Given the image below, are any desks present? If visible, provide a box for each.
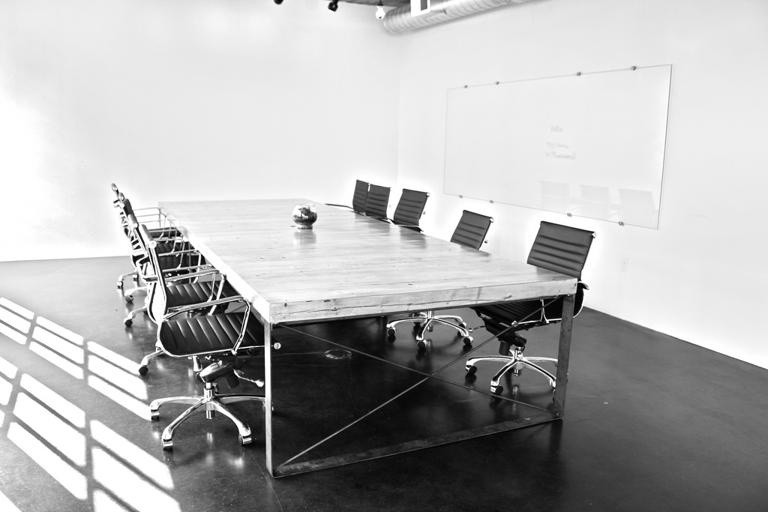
[156,200,577,479]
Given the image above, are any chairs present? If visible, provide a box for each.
[327,180,429,234]
[465,220,594,395]
[383,207,495,351]
[110,180,240,382]
[109,179,594,454]
[140,226,277,449]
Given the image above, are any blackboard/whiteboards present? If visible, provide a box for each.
[442,63,671,230]
